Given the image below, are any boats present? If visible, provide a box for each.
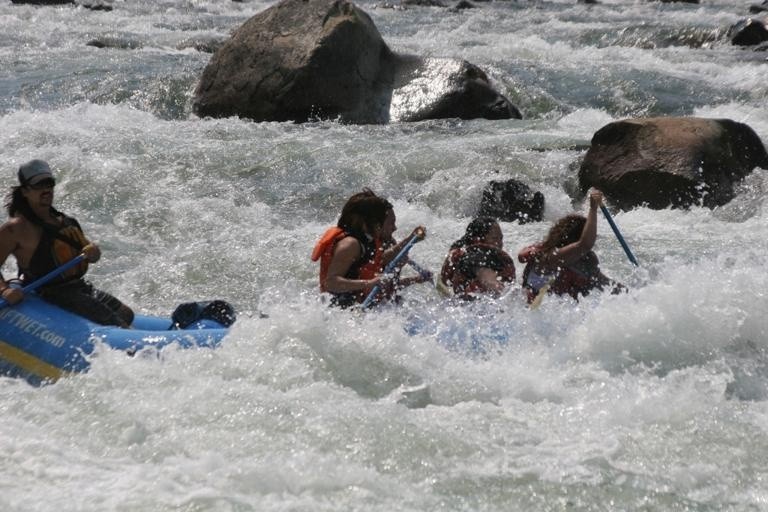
[0,276,507,388]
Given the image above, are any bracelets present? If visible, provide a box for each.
[1,286,9,295]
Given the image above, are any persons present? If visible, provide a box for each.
[0,159,135,330]
[516,188,628,310]
[361,196,434,304]
[442,217,505,296]
[310,186,423,311]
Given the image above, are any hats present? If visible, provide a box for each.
[17,160,56,185]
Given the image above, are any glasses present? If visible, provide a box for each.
[27,179,55,190]
